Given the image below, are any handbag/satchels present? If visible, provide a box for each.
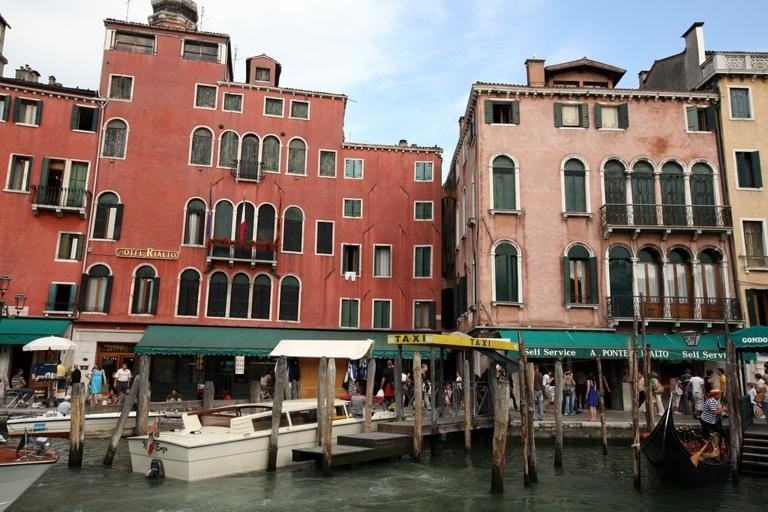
[425,382,429,390]
[653,383,662,393]
[673,387,682,396]
[753,391,764,404]
[566,390,570,395]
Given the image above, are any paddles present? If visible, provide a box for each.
[689,437,712,466]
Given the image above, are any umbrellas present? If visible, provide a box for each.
[21,334,80,383]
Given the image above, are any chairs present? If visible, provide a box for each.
[2,376,32,409]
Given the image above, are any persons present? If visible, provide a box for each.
[342,355,464,411]
[112,363,133,397]
[166,387,182,402]
[637,366,768,449]
[64,362,81,395]
[55,394,71,415]
[89,361,106,406]
[84,366,93,405]
[55,360,67,378]
[222,390,232,400]
[10,367,34,404]
[507,365,612,421]
[109,388,117,399]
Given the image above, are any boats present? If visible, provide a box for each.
[128,397,396,481]
[7,411,166,439]
[0,437,60,512]
[637,390,731,493]
